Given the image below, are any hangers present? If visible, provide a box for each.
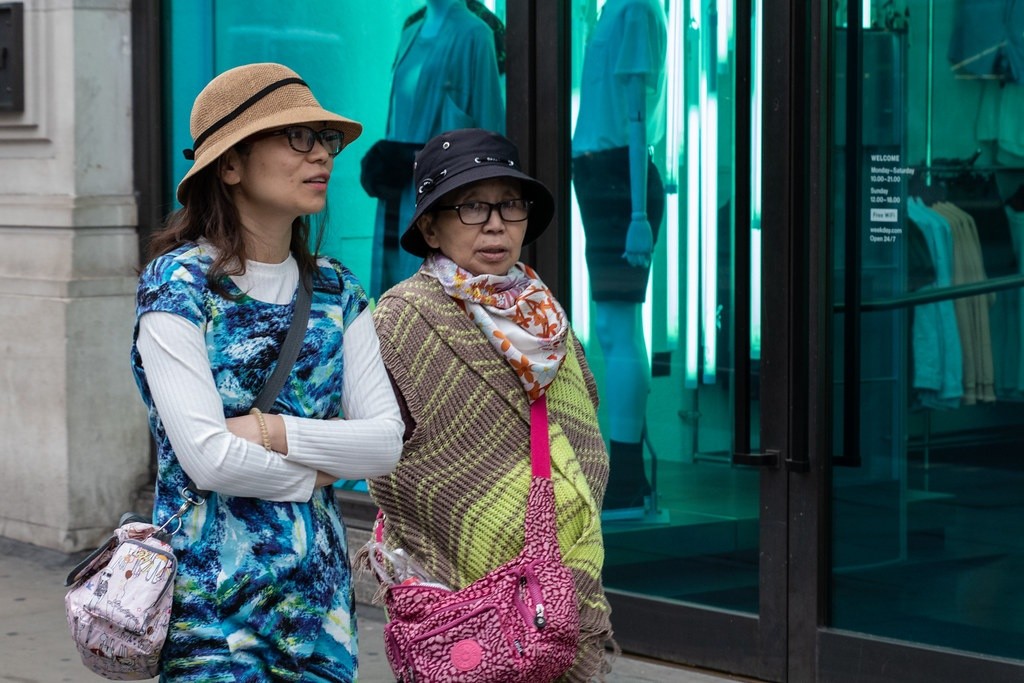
[908,164,995,209]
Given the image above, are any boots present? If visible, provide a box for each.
[596,437,650,521]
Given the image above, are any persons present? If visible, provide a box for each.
[367,133,615,683]
[567,0,672,519]
[130,62,405,683]
[373,0,503,293]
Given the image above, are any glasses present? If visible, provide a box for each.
[430,198,533,226]
[236,125,345,159]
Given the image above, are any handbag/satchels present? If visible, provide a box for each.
[357,138,425,199]
[384,548,581,683]
[64,520,178,680]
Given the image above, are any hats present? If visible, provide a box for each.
[399,128,555,259]
[177,62,364,209]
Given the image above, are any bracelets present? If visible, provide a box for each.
[250,407,271,453]
